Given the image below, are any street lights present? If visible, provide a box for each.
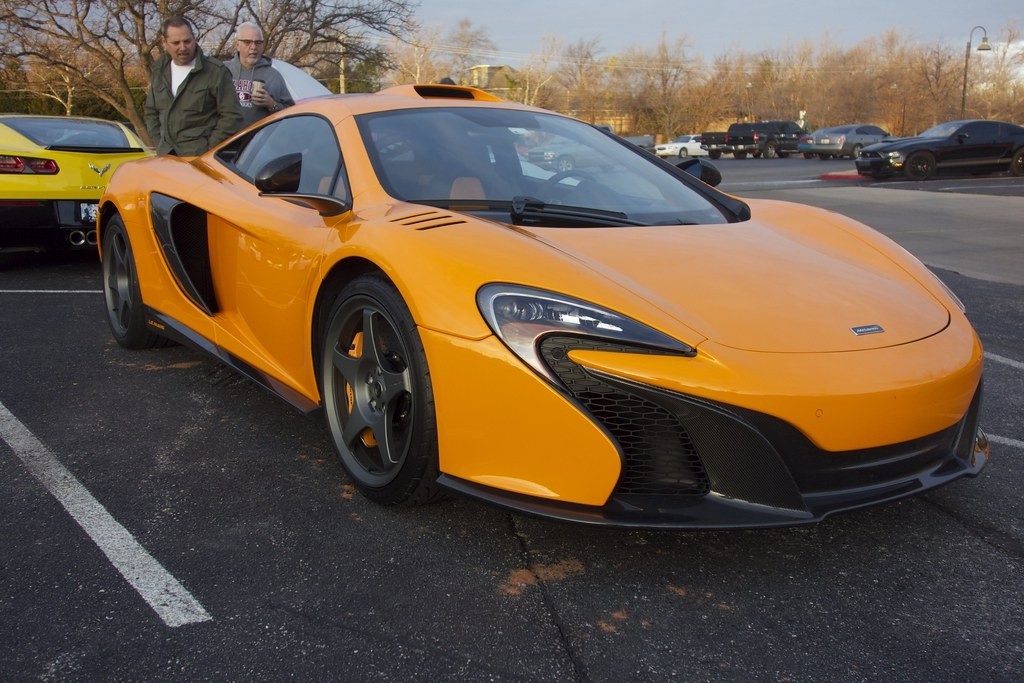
[961,25,991,119]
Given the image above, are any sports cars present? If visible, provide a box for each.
[0,111,155,259]
[97,82,991,530]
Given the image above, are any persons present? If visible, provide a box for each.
[222,22,296,127]
[145,17,242,158]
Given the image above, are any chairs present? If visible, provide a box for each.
[419,139,515,199]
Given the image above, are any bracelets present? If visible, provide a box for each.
[268,103,278,111]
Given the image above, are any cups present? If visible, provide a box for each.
[252,79,265,103]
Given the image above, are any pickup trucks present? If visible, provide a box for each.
[701,120,805,158]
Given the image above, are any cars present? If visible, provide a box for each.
[655,134,711,158]
[855,120,1024,180]
[798,123,893,160]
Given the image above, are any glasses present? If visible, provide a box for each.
[237,39,264,45]
